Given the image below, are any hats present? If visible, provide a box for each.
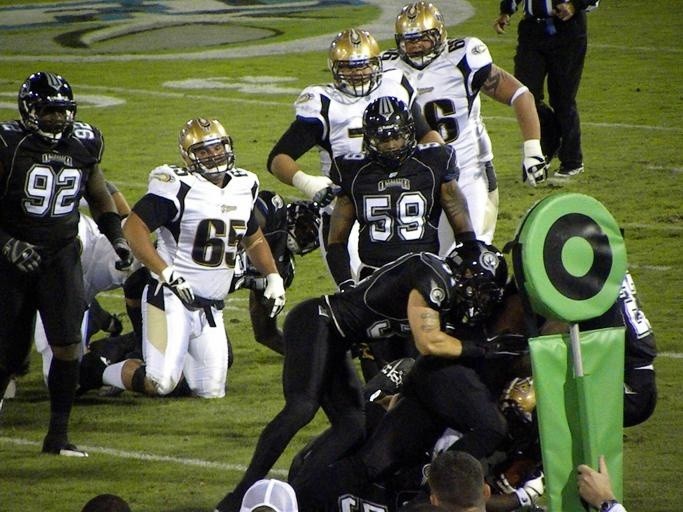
[239,479,299,512]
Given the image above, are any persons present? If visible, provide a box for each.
[207,241,509,509]
[85,183,325,383]
[267,28,453,293]
[114,115,286,400]
[570,458,631,512]
[494,1,598,176]
[321,94,476,295]
[0,73,134,459]
[427,448,494,511]
[308,345,565,512]
[380,8,549,265]
[589,232,660,429]
[37,175,147,391]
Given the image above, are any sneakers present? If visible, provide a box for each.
[89,335,129,364]
[98,386,125,399]
[541,141,562,164]
[43,436,89,458]
[552,157,585,178]
[75,354,110,398]
[216,493,240,512]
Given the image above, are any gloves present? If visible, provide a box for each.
[3,240,43,275]
[151,266,196,305]
[477,330,529,362]
[514,467,546,508]
[293,170,342,208]
[261,273,287,321]
[105,228,136,271]
[520,139,548,187]
[339,279,357,295]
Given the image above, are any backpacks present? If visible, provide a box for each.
[511,203,657,469]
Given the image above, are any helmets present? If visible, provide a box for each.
[287,201,335,256]
[363,97,417,171]
[179,118,235,175]
[18,72,78,145]
[499,376,537,435]
[395,2,447,70]
[328,30,382,97]
[445,240,508,321]
